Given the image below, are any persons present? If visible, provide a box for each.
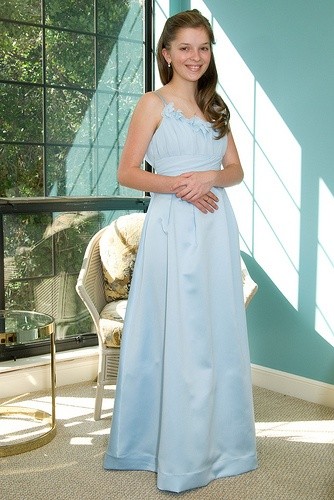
[104,6,260,495]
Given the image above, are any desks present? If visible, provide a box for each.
[0,305,60,459]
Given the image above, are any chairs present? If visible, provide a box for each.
[75,209,260,424]
